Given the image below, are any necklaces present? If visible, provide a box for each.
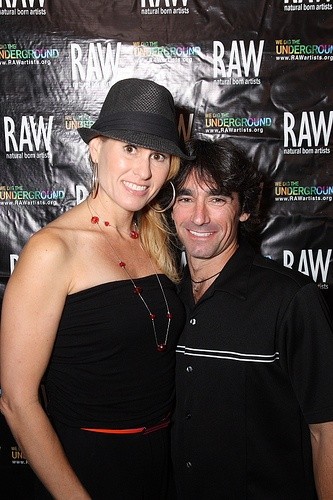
[89,196,138,239]
[86,195,173,351]
[191,272,220,292]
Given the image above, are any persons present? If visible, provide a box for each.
[156,137,333,500]
[0,78,196,500]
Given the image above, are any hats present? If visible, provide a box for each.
[76,78,195,161]
